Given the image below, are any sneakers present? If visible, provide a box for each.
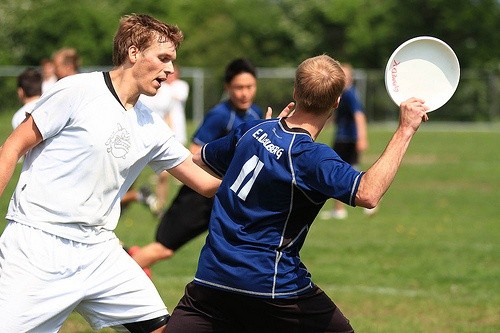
[364,207,377,214]
[335,208,347,220]
[139,187,161,216]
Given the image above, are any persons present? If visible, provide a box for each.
[119,62,190,215]
[1,13,295,333]
[320,64,378,219]
[162,55,429,333]
[123,58,266,278]
[12,47,80,132]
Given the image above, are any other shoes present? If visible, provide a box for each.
[128,244,152,277]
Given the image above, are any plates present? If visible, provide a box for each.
[384,37,460,113]
